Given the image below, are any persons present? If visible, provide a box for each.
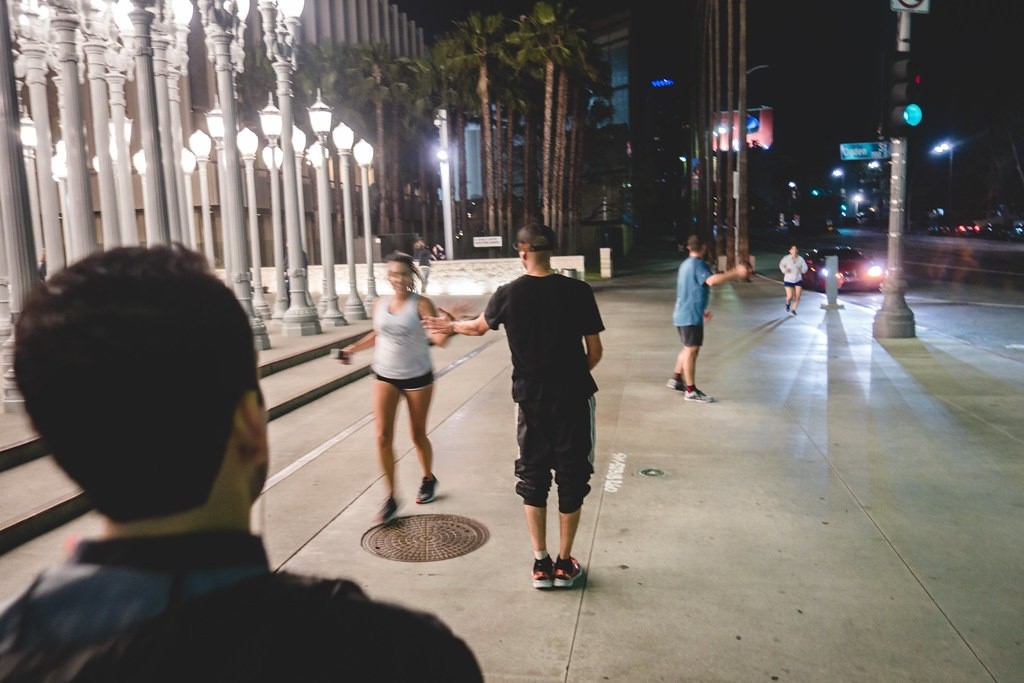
[340,250,451,520]
[419,246,437,293]
[779,244,808,315]
[283,240,308,307]
[421,223,606,589]
[666,234,748,403]
[0,239,487,683]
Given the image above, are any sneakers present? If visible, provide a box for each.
[666,379,687,392]
[553,557,582,587]
[377,497,401,522]
[684,390,714,403]
[416,473,439,504]
[532,559,556,588]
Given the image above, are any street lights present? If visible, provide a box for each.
[257,0,324,337]
[935,141,953,226]
[350,138,382,304]
[197,0,273,351]
[237,127,270,320]
[290,123,318,314]
[186,128,216,274]
[432,108,456,261]
[330,120,368,321]
[0,0,309,339]
[305,86,347,328]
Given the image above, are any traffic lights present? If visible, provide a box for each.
[882,50,924,139]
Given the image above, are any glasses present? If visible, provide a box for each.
[512,240,518,250]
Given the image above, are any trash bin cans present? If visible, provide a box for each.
[563,268,577,277]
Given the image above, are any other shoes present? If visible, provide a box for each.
[786,303,790,312]
[789,310,797,317]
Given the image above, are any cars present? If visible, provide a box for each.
[800,244,884,294]
